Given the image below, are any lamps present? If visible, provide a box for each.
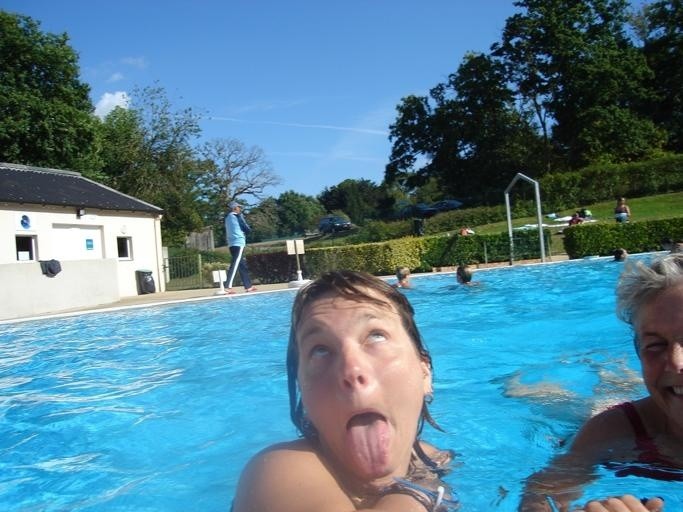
[76,208,86,217]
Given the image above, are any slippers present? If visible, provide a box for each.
[224,287,256,295]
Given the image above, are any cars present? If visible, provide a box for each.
[402,200,462,218]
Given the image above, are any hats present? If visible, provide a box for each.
[227,202,242,208]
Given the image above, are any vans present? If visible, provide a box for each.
[318,217,352,233]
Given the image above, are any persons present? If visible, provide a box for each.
[613,248,628,262]
[568,213,583,225]
[230,269,456,512]
[391,267,414,288]
[661,237,683,253]
[224,201,257,295]
[613,197,631,223]
[456,265,480,286]
[517,250,683,512]
